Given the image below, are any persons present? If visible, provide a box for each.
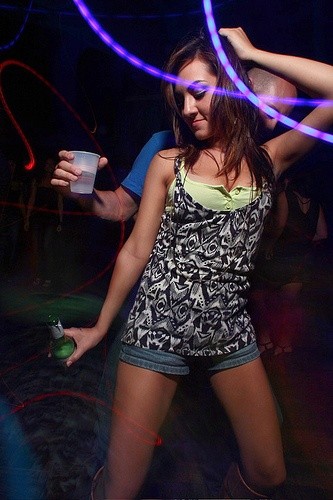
[0,66,333,460]
[49,27,333,500]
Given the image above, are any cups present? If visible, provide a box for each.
[69,151,101,194]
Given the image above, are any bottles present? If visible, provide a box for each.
[47,313,77,365]
